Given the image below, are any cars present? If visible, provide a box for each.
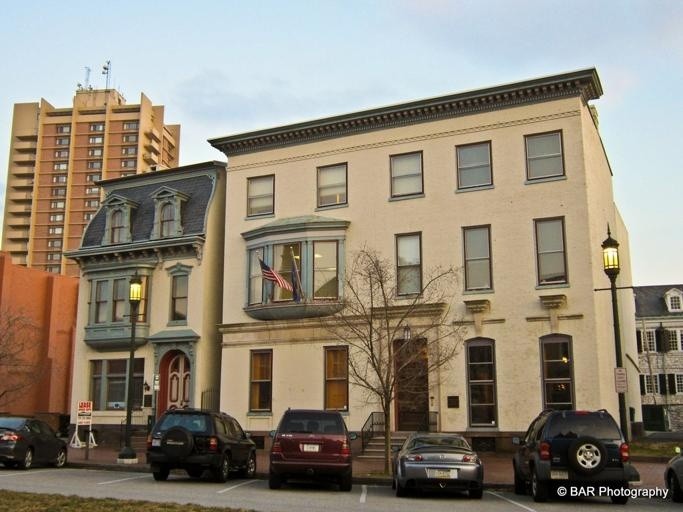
[0,413,67,468]
[388,432,487,500]
[663,446,683,500]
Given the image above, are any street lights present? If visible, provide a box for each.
[601,224,642,483]
[112,268,141,466]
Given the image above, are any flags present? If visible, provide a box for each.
[289,258,306,304]
[257,257,294,293]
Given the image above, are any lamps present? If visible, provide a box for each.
[402,323,411,342]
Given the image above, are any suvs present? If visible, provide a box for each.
[141,406,261,483]
[267,406,356,490]
[511,408,633,504]
